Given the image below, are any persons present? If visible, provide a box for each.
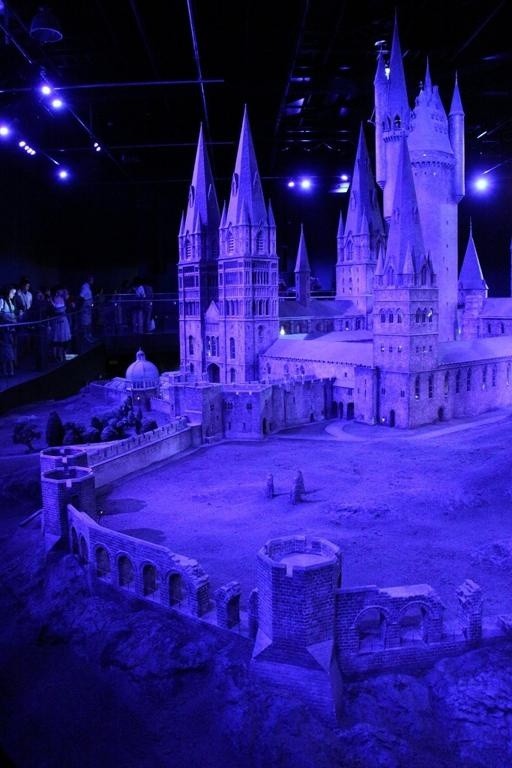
[265,471,277,500]
[1,271,158,373]
[289,465,307,506]
